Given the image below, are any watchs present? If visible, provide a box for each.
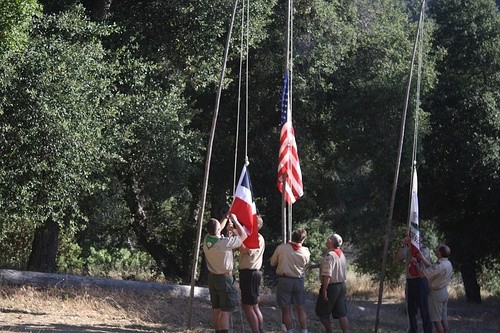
[413,262,418,266]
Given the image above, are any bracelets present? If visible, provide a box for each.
[232,219,238,225]
[399,241,404,248]
[225,213,230,219]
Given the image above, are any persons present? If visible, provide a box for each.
[227,215,266,333]
[306,233,350,333]
[396,232,433,333]
[203,208,248,333]
[270,228,310,333]
[410,244,453,333]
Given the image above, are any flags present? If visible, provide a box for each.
[227,160,260,249]
[407,161,423,276]
[277,68,304,205]
